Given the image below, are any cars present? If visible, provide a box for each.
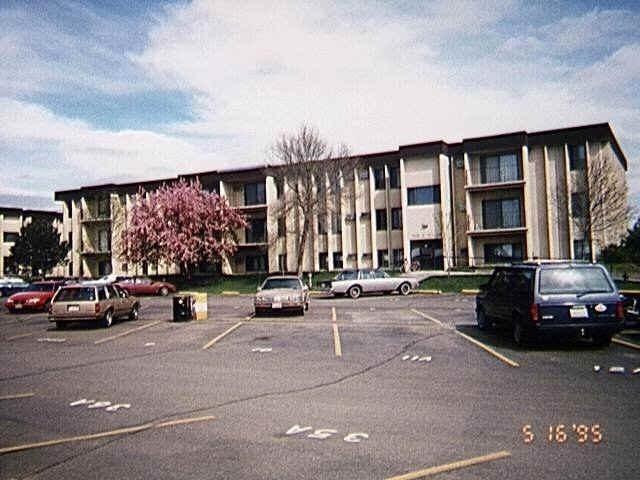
[254,276,309,316]
[0,277,176,329]
[317,269,417,298]
[475,262,626,346]
